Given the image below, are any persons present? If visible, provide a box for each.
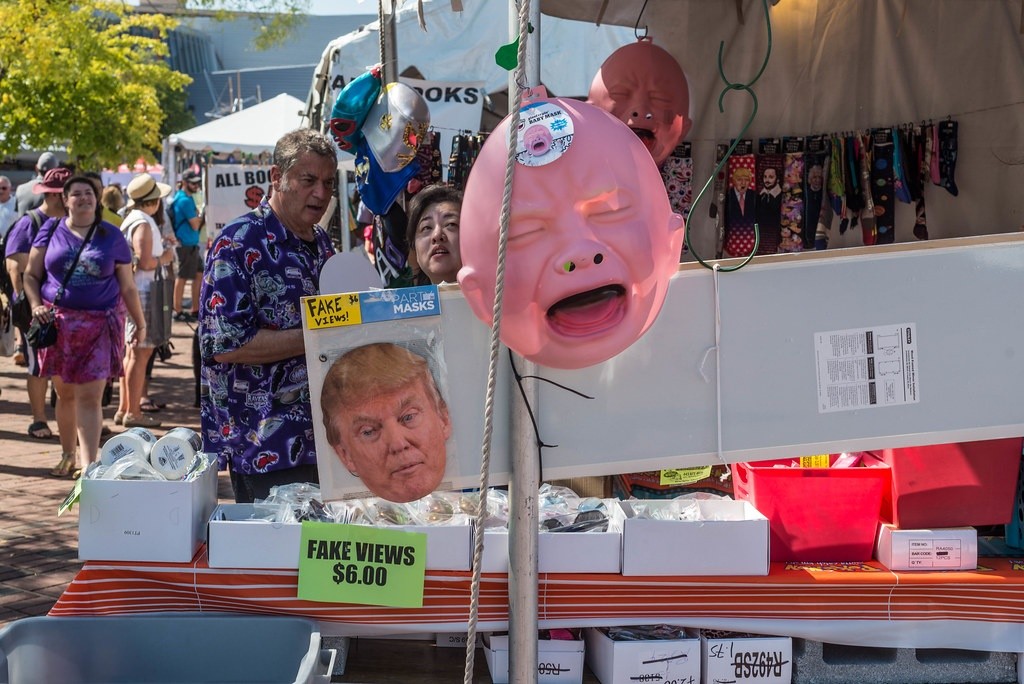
[586,36,691,165]
[0,152,204,479]
[321,343,452,502]
[409,184,462,285]
[457,84,685,371]
[195,129,339,505]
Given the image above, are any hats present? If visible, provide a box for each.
[101,186,125,208]
[354,137,422,214]
[36,152,58,170]
[330,65,382,155]
[182,169,201,182]
[127,173,172,200]
[32,168,74,194]
[361,81,431,172]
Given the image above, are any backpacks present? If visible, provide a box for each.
[167,196,187,233]
[0,210,41,293]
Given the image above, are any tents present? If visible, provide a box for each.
[170,91,358,254]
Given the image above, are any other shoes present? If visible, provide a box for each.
[13,349,25,364]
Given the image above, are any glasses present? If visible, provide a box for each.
[0,186,9,190]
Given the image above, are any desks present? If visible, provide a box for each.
[48,557,1024,683]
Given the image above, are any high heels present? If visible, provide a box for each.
[50,452,75,476]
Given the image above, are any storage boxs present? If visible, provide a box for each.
[478,500,621,578]
[79,453,218,563]
[875,524,979,574]
[592,627,700,684]
[616,498,770,576]
[703,631,793,684]
[324,501,473,574]
[477,630,583,683]
[733,452,891,565]
[1,616,337,684]
[203,502,308,568]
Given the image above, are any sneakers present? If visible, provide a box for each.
[123,415,161,427]
[114,414,123,424]
[174,312,195,322]
[191,312,199,319]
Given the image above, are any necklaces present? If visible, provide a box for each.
[70,221,92,228]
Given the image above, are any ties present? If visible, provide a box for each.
[739,192,745,215]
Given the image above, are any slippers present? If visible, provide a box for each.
[28,422,52,439]
[141,399,160,412]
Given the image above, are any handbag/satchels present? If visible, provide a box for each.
[12,289,31,334]
[157,340,174,359]
[27,310,56,348]
[151,256,175,342]
